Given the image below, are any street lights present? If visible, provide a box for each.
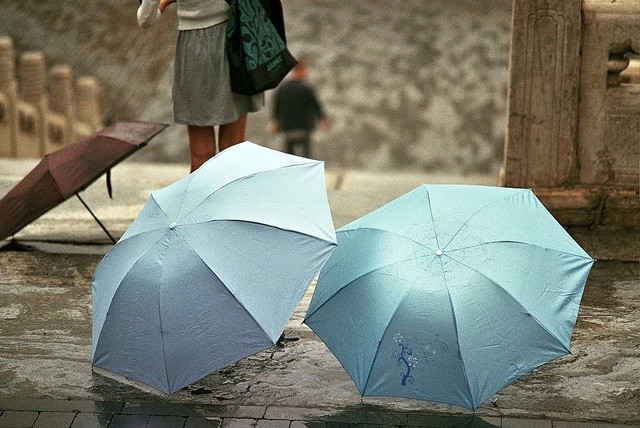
[226,1,297,97]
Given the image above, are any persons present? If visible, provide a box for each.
[268,58,330,158]
[136,1,265,175]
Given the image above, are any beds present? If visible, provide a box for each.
[1,119,170,245]
[89,141,340,397]
[301,183,598,413]
